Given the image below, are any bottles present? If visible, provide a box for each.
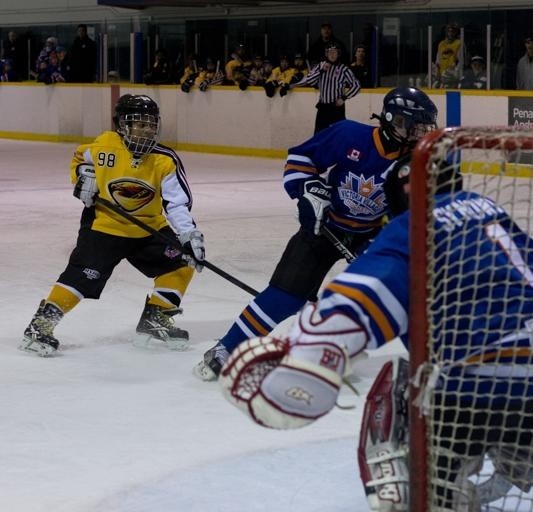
[407,76,423,89]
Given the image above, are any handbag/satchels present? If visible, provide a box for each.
[182,232,206,272]
[74,175,98,207]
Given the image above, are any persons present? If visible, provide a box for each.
[243,156,533,512]
[192,83,438,381]
[1,17,380,97]
[426,20,533,90]
[302,41,361,135]
[22,93,205,356]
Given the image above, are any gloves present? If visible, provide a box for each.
[290,72,303,84]
[280,83,291,97]
[239,79,248,90]
[296,178,332,240]
[265,80,277,97]
[222,79,235,87]
[181,79,194,93]
[200,79,212,90]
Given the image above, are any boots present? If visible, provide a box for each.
[24,300,63,350]
[137,295,190,338]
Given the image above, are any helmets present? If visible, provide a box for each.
[47,38,57,47]
[324,43,341,58]
[380,86,442,147]
[114,94,162,158]
[383,154,464,217]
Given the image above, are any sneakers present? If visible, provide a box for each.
[204,343,230,376]
